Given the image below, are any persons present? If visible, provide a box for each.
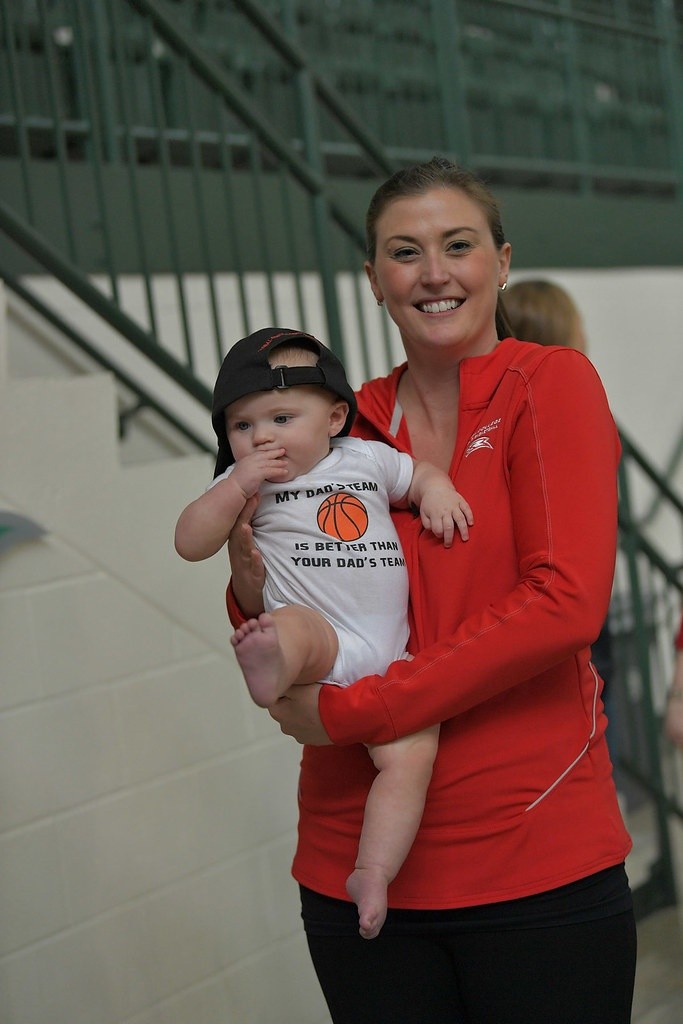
[665,617,683,747]
[175,327,475,941]
[501,280,591,356]
[225,155,637,1024]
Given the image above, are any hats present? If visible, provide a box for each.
[211,327,358,483]
